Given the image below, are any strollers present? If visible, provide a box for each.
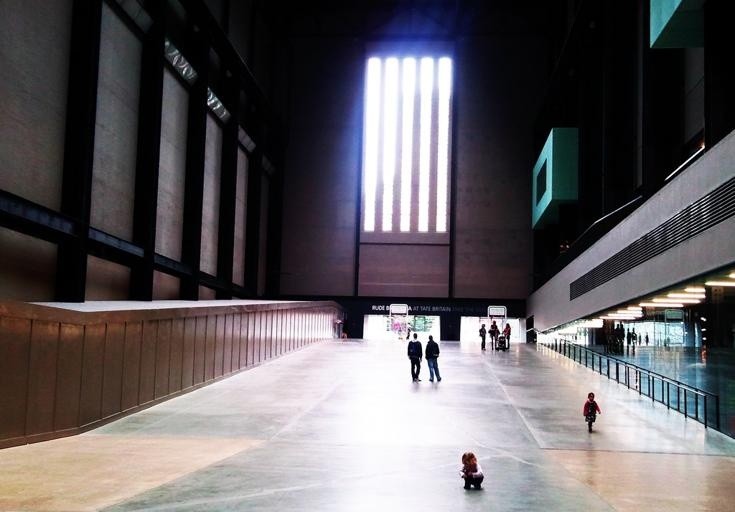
[496,334,506,351]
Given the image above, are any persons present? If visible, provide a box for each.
[479,323,486,350]
[582,392,603,433]
[488,321,501,350]
[407,332,423,382]
[503,323,511,349]
[457,452,485,490]
[638,332,642,345]
[602,322,638,356]
[425,335,442,381]
[646,331,649,346]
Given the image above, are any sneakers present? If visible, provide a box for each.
[482,344,511,351]
[464,484,482,491]
[589,427,592,433]
[413,378,441,382]
